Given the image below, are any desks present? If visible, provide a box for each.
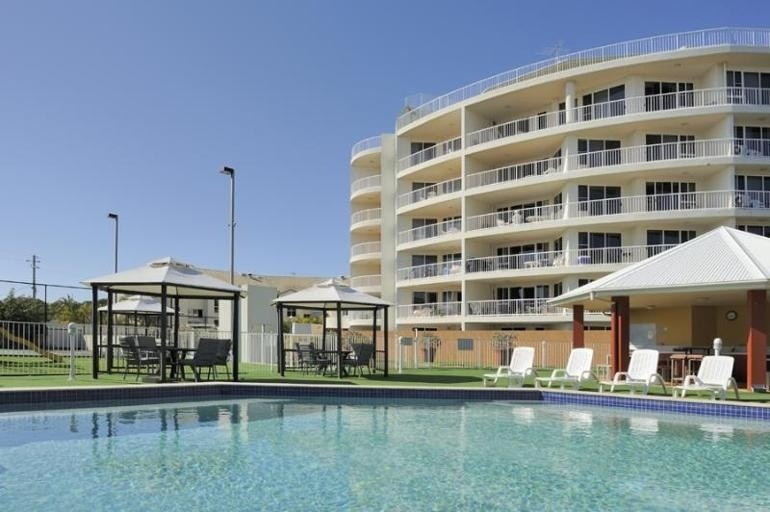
[673,356,740,401]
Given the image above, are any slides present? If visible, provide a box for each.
[1,328,63,362]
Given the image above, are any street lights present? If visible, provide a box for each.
[107,214,117,308]
[218,165,235,285]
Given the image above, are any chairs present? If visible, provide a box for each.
[599,349,667,396]
[535,348,599,390]
[484,346,543,388]
[118,336,231,382]
[295,341,375,379]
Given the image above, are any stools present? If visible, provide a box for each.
[687,355,702,375]
[670,355,686,385]
